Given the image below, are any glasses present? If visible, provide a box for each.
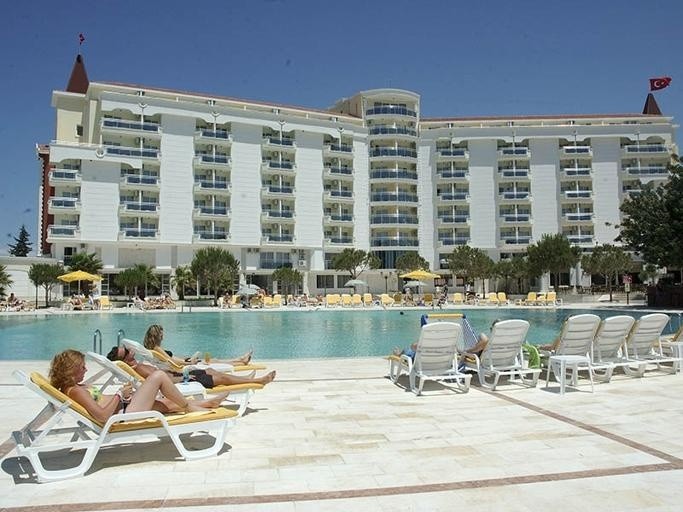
[121,347,129,361]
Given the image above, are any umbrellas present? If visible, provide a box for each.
[403,281,428,294]
[57,270,104,295]
[344,280,368,294]
[398,268,480,308]
[236,288,258,302]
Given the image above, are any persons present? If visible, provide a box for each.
[144,325,252,365]
[535,313,576,350]
[288,293,325,305]
[400,311,404,316]
[69,292,94,310]
[48,349,230,423]
[134,295,174,311]
[107,347,276,388]
[8,293,26,307]
[223,289,266,309]
[393,341,418,363]
[537,294,551,307]
[457,319,502,360]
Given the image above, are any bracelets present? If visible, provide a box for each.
[116,388,131,403]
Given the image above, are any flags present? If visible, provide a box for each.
[648,77,672,91]
[79,33,85,45]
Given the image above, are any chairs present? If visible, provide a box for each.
[216,296,231,307]
[326,295,340,309]
[120,339,266,378]
[87,351,264,420]
[264,297,273,307]
[628,313,682,377]
[487,292,497,302]
[498,292,506,303]
[528,293,536,304]
[425,295,434,307]
[380,294,395,307]
[653,325,683,374]
[463,320,542,390]
[454,294,462,304]
[101,296,110,309]
[388,322,472,395]
[287,294,324,306]
[274,294,282,305]
[12,366,239,483]
[592,316,644,382]
[341,294,352,308]
[537,292,546,303]
[132,296,174,309]
[547,293,556,302]
[231,294,261,308]
[363,293,372,307]
[61,294,100,310]
[354,294,361,306]
[541,314,601,394]
